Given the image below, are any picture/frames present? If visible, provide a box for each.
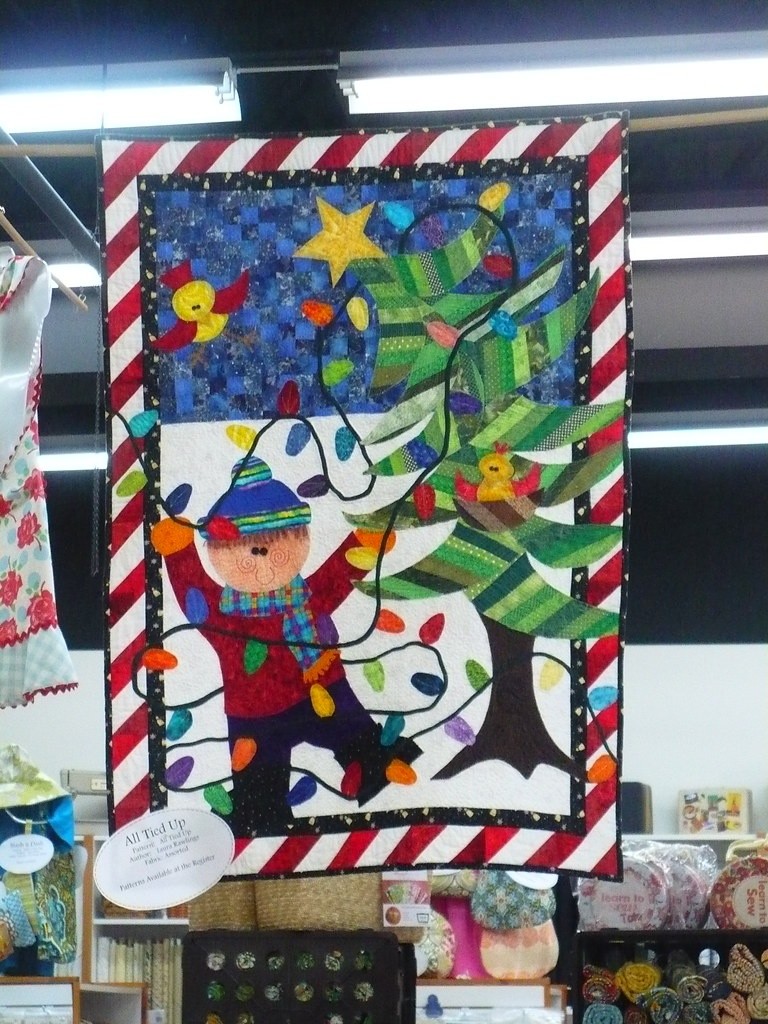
[677,786,753,835]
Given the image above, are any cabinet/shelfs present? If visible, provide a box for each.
[0,834,189,1024]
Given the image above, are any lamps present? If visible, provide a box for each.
[335,26,768,121]
[0,57,245,146]
